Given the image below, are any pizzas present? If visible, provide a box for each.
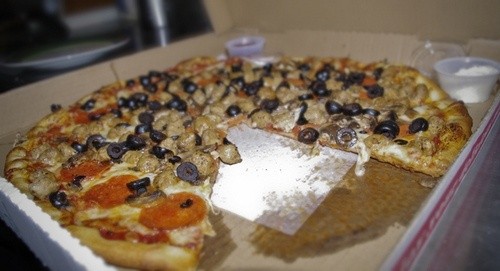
[4,53,474,271]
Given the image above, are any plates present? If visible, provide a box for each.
[3,35,131,69]
